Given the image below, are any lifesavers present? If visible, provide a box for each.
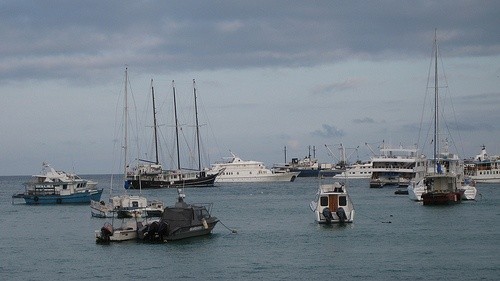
[56,198,62,204]
[34,197,38,201]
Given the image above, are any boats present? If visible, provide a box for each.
[10,162,105,206]
[309,180,356,227]
[273,143,500,187]
[208,150,302,183]
[93,178,221,244]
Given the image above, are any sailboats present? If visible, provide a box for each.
[90,62,165,218]
[125,77,219,188]
[407,25,478,205]
